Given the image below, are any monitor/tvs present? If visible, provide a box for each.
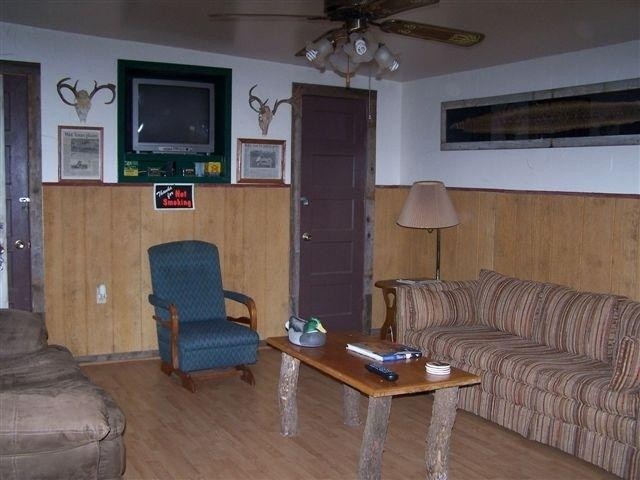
[134,78,215,156]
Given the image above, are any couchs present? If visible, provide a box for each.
[0,303,128,480]
[397,267,640,480]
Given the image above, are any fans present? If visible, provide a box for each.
[208,0,487,57]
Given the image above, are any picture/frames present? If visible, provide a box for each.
[56,124,105,181]
[437,71,640,151]
[237,136,287,184]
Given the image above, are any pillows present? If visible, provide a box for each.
[474,267,543,340]
[608,336,640,391]
[533,284,628,369]
[407,284,477,331]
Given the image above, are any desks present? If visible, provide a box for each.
[375,279,399,342]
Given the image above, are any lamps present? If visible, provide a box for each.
[302,25,400,83]
[392,179,460,281]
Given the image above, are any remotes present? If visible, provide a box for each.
[366,360,399,384]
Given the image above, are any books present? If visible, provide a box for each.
[345,339,422,363]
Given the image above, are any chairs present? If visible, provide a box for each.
[147,237,259,395]
